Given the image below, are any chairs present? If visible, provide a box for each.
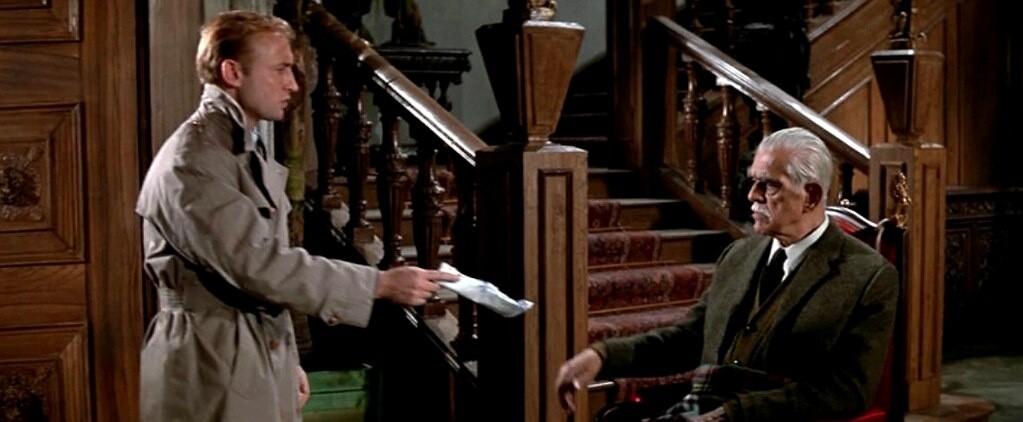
[824,205,897,421]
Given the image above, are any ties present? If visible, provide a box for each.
[759,249,788,308]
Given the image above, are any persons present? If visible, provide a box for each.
[135,9,458,422]
[557,127,897,422]
